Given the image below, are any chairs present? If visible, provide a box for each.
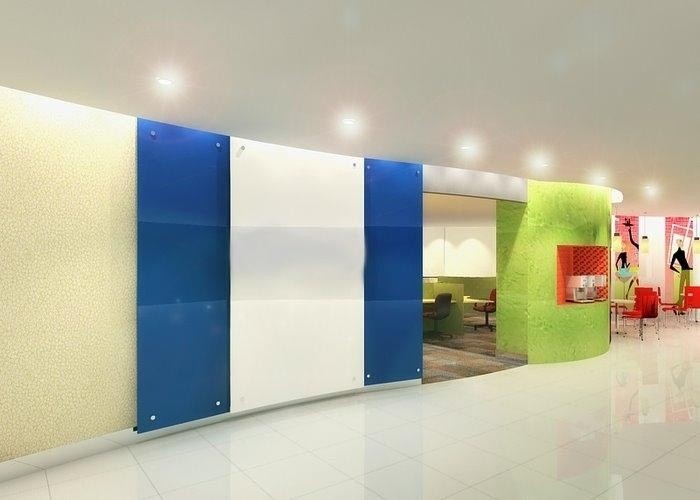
[473,288,496,333]
[610,284,700,342]
[423,292,454,341]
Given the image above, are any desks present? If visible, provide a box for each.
[423,299,457,305]
[463,298,495,304]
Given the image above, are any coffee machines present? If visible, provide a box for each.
[566,275,607,303]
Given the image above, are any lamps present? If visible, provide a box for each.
[612,207,621,253]
[639,212,649,255]
[693,215,700,255]
